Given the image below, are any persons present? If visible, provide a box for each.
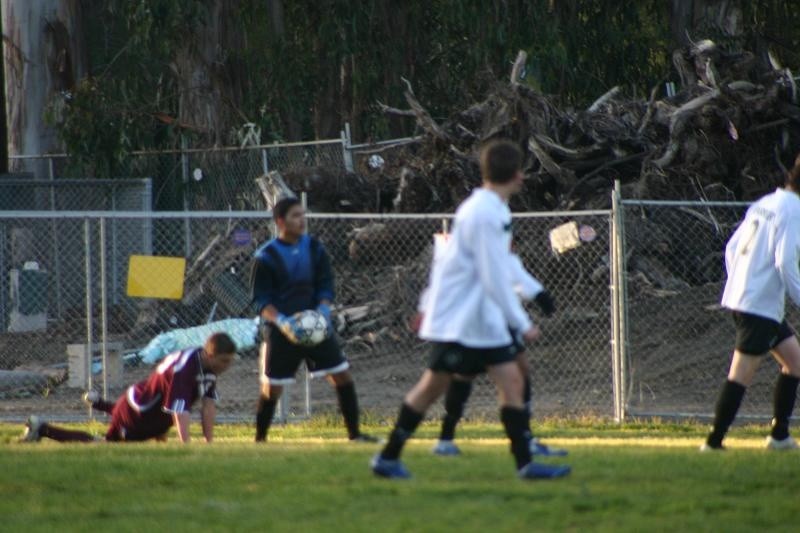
[372,140,574,480]
[249,197,378,445]
[21,332,240,446]
[703,157,800,456]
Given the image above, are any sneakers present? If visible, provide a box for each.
[372,453,412,477]
[433,441,460,455]
[529,439,568,456]
[518,461,570,481]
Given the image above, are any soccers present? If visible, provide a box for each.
[291,309,326,347]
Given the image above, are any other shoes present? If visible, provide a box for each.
[24,414,44,443]
[350,433,378,442]
[765,435,799,450]
[700,443,727,454]
[85,393,97,403]
